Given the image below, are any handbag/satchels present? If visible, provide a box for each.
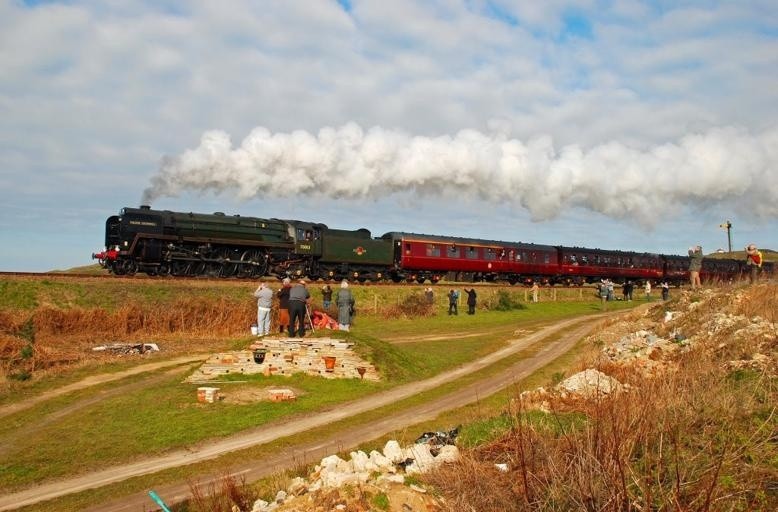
[452,300,456,303]
[349,305,356,314]
[751,256,760,262]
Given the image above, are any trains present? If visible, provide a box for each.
[92,205,778,288]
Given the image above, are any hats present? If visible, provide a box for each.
[282,278,291,283]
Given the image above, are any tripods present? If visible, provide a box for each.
[297,301,315,334]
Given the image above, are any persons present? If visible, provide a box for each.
[464,288,477,314]
[447,289,458,315]
[596,279,669,304]
[276,277,310,338]
[744,244,763,286]
[254,282,273,336]
[688,246,703,289]
[320,279,355,333]
[425,287,433,305]
[528,282,540,303]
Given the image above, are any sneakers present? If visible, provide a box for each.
[258,333,269,337]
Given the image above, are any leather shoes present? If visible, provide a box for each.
[289,333,306,336]
